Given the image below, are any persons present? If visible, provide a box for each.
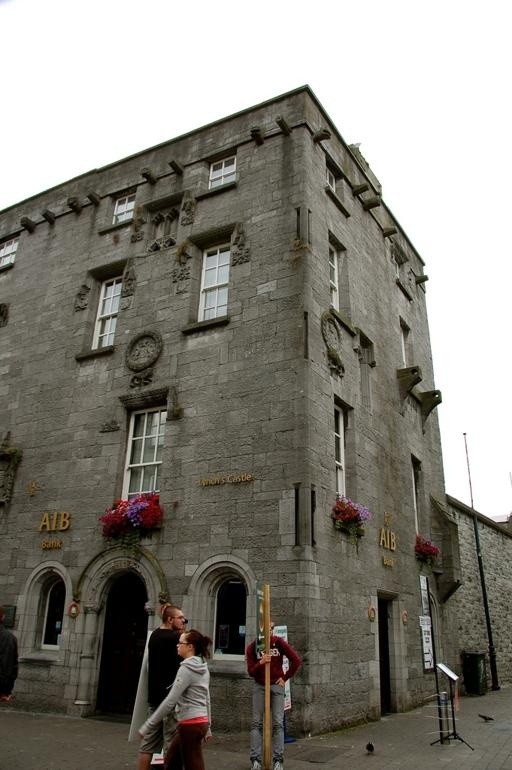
[0,607,18,703]
[246,617,300,769]
[137,629,212,770]
[138,607,186,769]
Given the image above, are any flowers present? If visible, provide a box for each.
[329,492,373,544]
[96,487,165,539]
[413,533,440,574]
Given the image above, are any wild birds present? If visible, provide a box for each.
[366,741,374,755]
[478,713,494,723]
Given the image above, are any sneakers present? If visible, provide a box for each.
[272,760,285,770]
[249,759,263,770]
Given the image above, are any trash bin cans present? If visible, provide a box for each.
[460,650,487,696]
